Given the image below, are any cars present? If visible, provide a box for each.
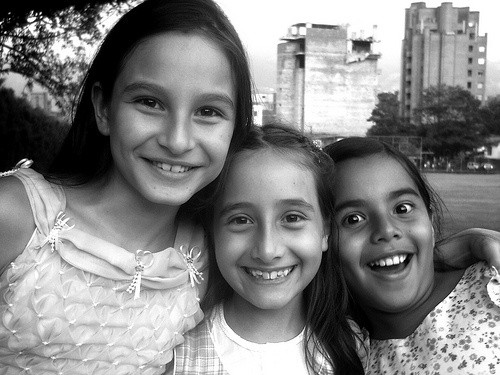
[467,154,494,173]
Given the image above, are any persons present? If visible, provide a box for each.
[313,136,499,375]
[0,0,499,375]
[162,124,350,375]
